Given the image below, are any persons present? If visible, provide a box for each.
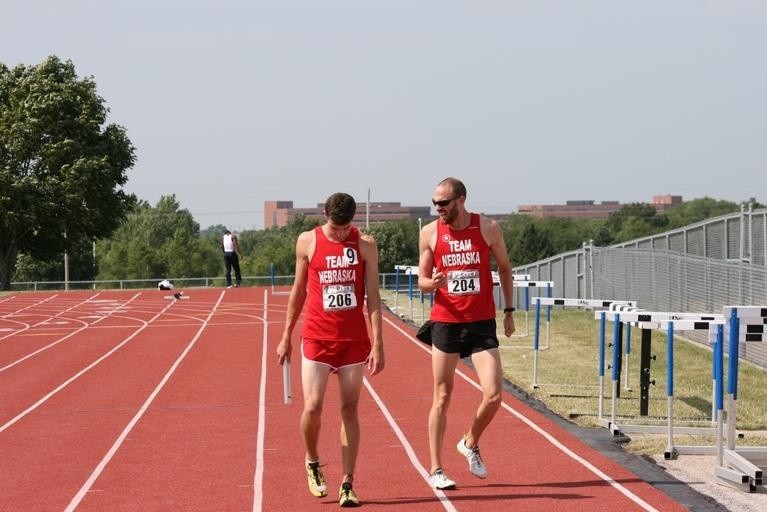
[276,190,384,508]
[220,229,244,290]
[411,175,516,490]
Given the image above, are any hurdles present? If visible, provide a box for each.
[594,306,767,493]
[394,264,554,351]
[530,296,638,392]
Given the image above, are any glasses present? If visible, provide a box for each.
[431,195,458,207]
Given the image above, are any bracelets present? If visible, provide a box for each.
[501,306,516,314]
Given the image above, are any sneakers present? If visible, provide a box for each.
[234,281,240,287]
[429,467,456,491]
[305,458,328,499]
[456,438,488,479]
[338,482,360,507]
[227,284,232,289]
